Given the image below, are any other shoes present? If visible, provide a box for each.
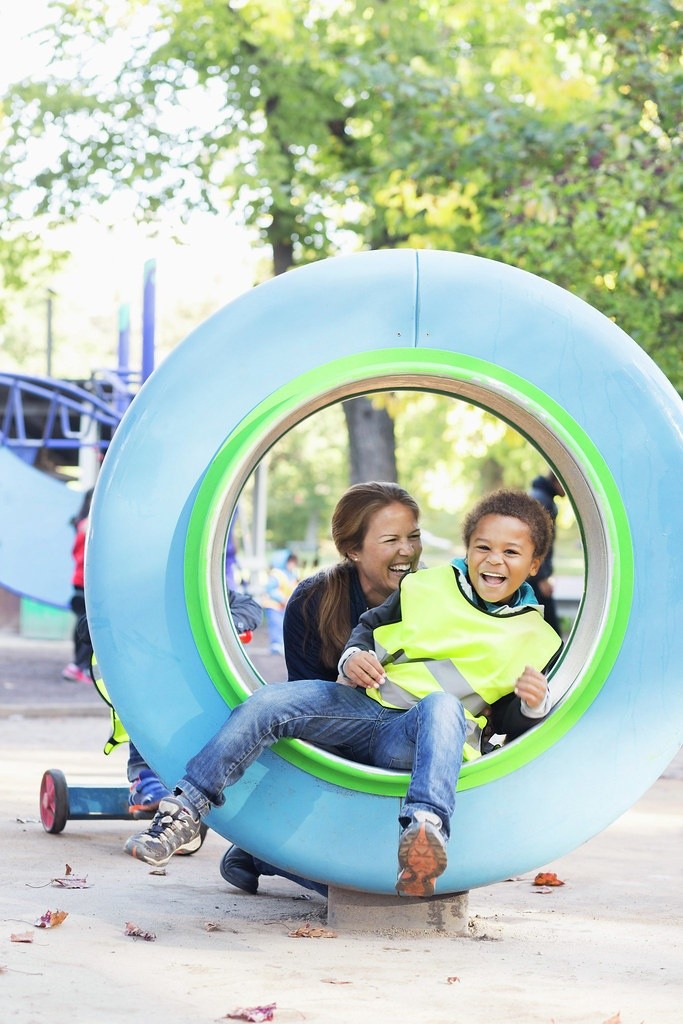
[61,663,92,684]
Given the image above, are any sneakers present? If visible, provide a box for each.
[123,797,201,867]
[220,844,260,894]
[395,810,448,898]
[128,769,172,811]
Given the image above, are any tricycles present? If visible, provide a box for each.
[37,630,252,854]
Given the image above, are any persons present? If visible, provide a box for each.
[76,574,263,812]
[122,487,565,897]
[528,472,567,643]
[221,480,493,897]
[259,549,303,657]
[62,488,93,684]
